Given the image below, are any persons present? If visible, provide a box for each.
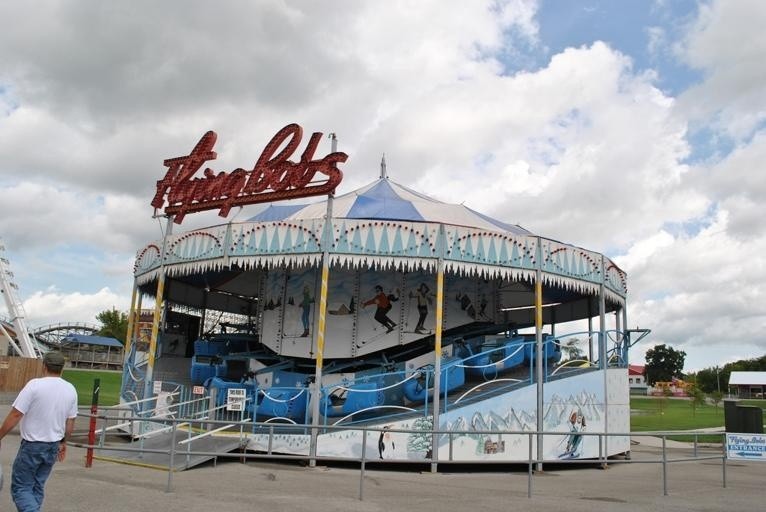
[479,293,488,315]
[461,294,476,319]
[413,283,433,333]
[566,410,581,456]
[0,352,78,511]
[299,286,315,337]
[361,285,397,334]
